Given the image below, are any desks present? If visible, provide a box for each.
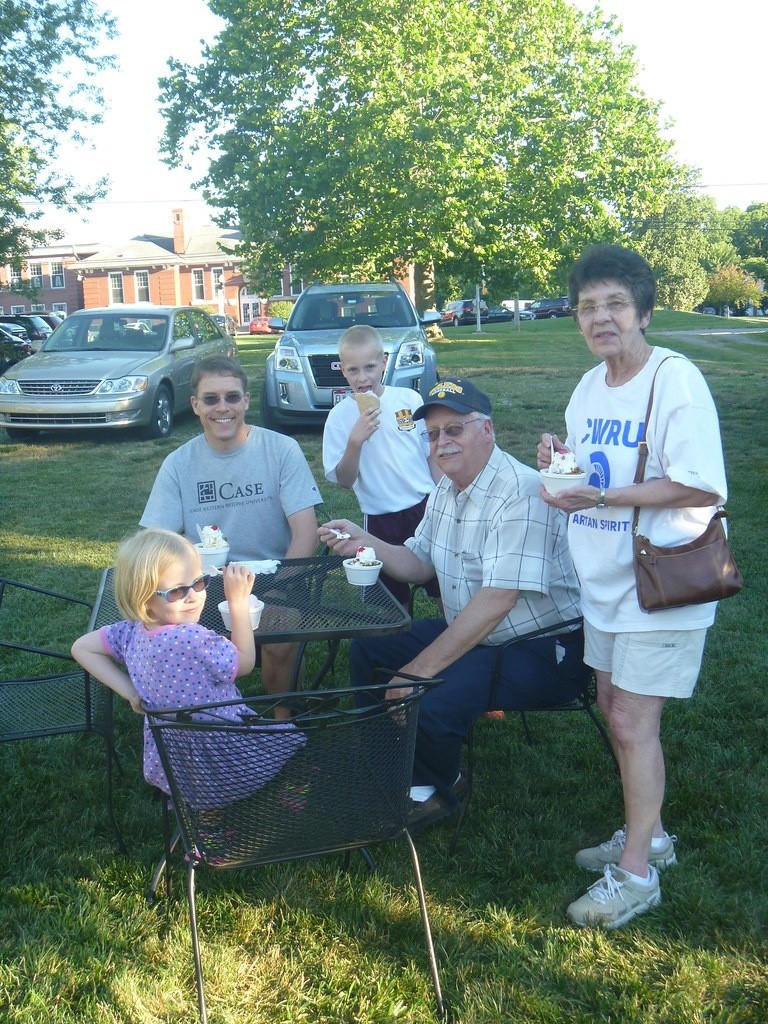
[85,551,415,899]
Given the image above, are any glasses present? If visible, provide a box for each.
[420,418,484,442]
[572,297,638,316]
[195,391,248,406]
[153,571,211,602]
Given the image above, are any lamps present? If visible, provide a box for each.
[76,275,86,282]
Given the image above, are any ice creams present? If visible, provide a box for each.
[348,546,382,566]
[250,595,260,609]
[196,523,228,548]
[546,448,586,476]
[355,390,380,415]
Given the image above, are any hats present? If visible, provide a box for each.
[411,377,492,422]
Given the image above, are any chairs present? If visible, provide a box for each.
[448,614,621,859]
[146,666,444,1024]
[369,297,401,326]
[151,322,167,348]
[254,507,339,692]
[312,301,341,328]
[99,320,119,338]
[0,577,125,855]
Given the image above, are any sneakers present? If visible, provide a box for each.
[574,826,679,871]
[567,860,661,928]
[184,829,233,865]
[278,779,311,812]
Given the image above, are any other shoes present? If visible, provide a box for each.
[386,774,466,837]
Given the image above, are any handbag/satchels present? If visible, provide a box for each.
[632,510,744,612]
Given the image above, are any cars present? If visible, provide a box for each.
[488,305,514,323]
[506,307,537,321]
[67,325,100,342]
[0,328,31,375]
[249,316,279,335]
[124,322,152,335]
[0,322,31,348]
[0,301,239,439]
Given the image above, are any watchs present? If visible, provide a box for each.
[597,488,605,509]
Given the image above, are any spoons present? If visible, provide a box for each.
[547,435,557,474]
[320,526,351,539]
[207,565,224,577]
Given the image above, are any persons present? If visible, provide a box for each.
[317,378,592,843]
[138,355,326,721]
[322,325,445,622]
[71,529,321,866]
[537,244,729,930]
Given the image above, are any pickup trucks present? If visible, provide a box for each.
[703,307,717,315]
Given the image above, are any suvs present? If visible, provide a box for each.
[0,316,52,341]
[210,315,238,336]
[10,312,67,330]
[523,297,571,319]
[265,274,441,437]
[440,299,490,327]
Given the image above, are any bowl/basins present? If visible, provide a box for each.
[192,543,231,571]
[540,467,586,497]
[217,600,265,632]
[342,558,384,585]
